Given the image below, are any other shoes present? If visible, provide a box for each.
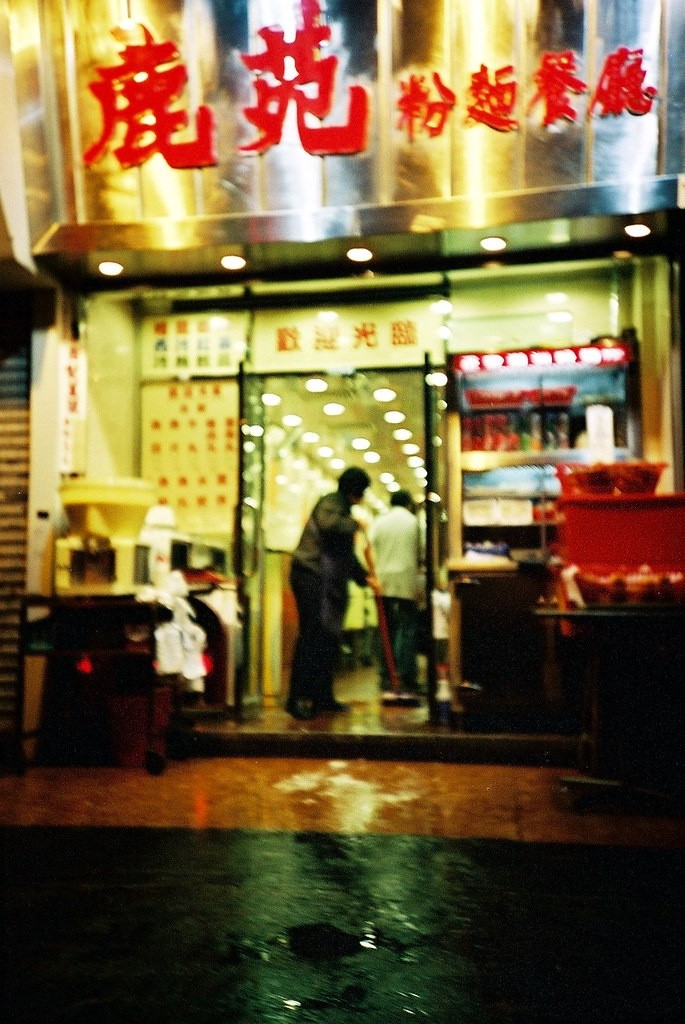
[284,700,306,720]
[312,701,343,712]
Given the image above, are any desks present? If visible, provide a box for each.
[12,584,224,778]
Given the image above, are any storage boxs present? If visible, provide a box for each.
[556,491,685,610]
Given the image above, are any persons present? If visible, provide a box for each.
[282,466,426,720]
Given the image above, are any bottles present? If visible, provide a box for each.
[531,413,544,452]
[544,413,558,451]
[521,414,531,452]
[462,412,521,452]
[559,413,569,450]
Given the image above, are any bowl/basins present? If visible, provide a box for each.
[570,464,616,495]
[618,464,670,490]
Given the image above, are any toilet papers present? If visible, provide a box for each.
[585,404,615,465]
